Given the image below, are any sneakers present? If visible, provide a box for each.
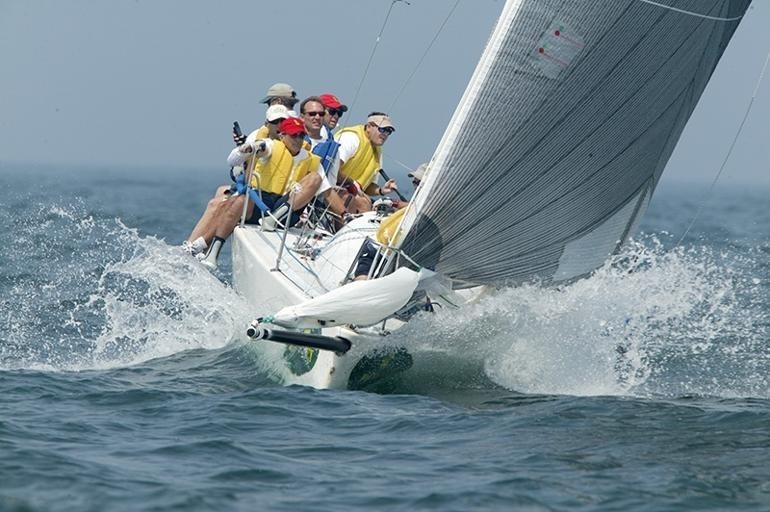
[341,211,363,224]
[193,248,206,262]
[178,240,198,258]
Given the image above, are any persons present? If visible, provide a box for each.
[351,161,431,283]
[198,117,350,273]
[185,80,395,261]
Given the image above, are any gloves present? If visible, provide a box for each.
[346,179,364,197]
[233,133,247,147]
[371,196,400,211]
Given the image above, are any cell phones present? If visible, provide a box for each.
[233,121,242,136]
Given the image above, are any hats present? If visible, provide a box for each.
[409,162,426,180]
[260,83,296,103]
[319,94,348,112]
[266,104,289,120]
[279,118,308,135]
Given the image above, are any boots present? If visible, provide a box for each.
[197,235,225,271]
[258,201,294,231]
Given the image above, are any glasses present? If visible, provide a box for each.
[326,108,343,117]
[304,111,326,115]
[269,119,287,124]
[290,133,305,138]
[378,127,393,133]
[412,179,421,186]
[265,99,277,106]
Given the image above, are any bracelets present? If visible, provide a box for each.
[341,210,348,218]
[379,188,384,196]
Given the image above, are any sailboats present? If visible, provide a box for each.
[231,0,752,391]
